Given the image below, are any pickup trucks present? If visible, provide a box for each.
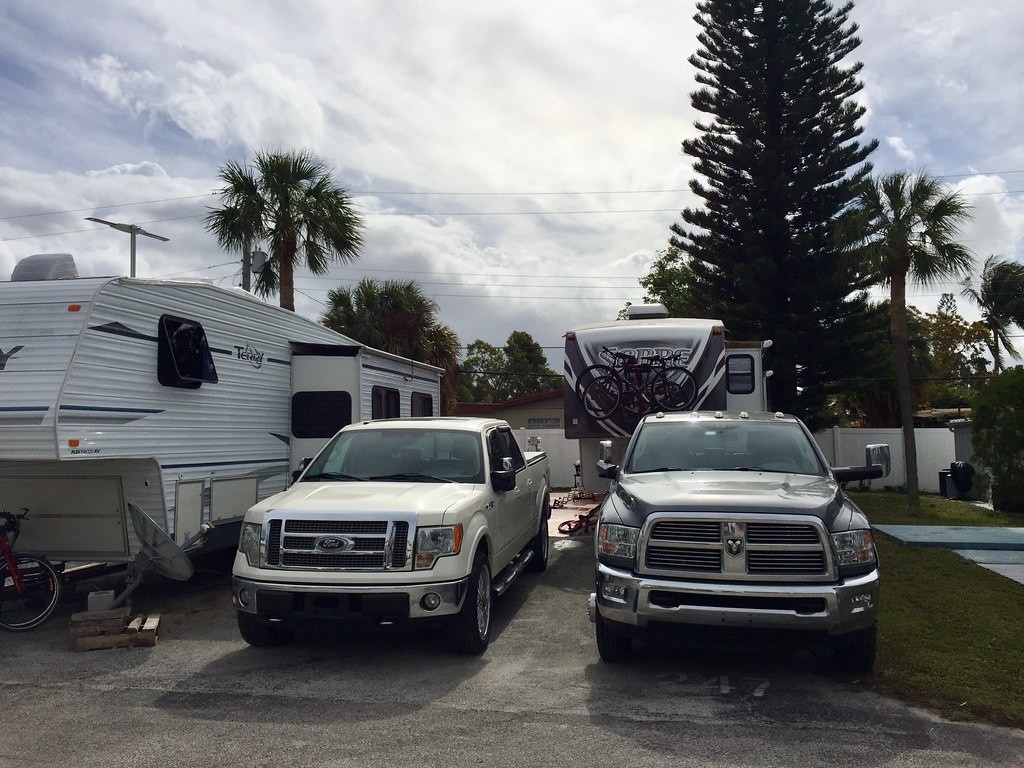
[232,417,551,654]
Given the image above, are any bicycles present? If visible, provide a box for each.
[575,346,698,420]
[0,508,62,632]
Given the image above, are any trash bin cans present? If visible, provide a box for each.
[939,468,962,498]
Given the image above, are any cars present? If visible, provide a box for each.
[588,410,891,665]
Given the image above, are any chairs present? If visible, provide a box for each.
[350,434,507,476]
[643,431,786,468]
